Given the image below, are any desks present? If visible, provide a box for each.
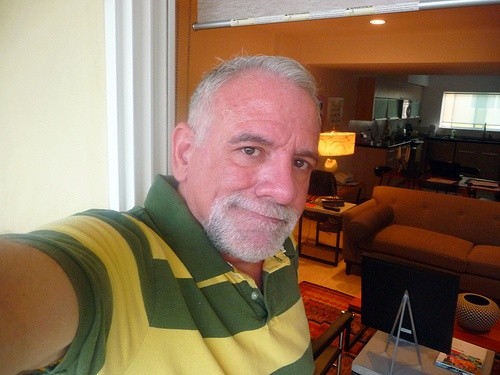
[372,167,500,202]
[352,329,495,375]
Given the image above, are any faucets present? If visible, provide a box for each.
[483,123,487,138]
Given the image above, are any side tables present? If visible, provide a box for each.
[298,198,357,267]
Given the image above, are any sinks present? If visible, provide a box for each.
[472,138,496,142]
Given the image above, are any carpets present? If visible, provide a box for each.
[298,280,500,375]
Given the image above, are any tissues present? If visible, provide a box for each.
[321,195,345,207]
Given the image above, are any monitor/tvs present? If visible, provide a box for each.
[347,117,400,144]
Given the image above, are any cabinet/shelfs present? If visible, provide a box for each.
[423,139,500,173]
[356,76,424,121]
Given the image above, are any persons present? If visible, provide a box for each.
[0,52,323,374]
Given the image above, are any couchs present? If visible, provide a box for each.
[342,185,500,303]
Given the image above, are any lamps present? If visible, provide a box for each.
[317,126,356,172]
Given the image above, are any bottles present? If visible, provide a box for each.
[450,127,456,139]
